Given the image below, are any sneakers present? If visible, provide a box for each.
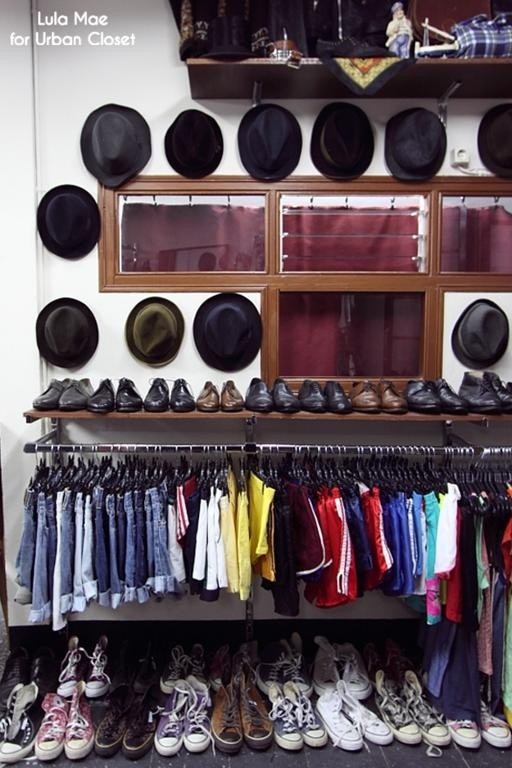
[0,630,511,764]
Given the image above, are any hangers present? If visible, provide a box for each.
[23,438,512,511]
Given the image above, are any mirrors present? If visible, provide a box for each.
[433,178,511,293]
[271,177,433,282]
[261,283,443,393]
[100,174,270,291]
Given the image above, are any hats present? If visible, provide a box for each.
[451,299,509,370]
[37,293,265,374]
[36,184,102,261]
[81,102,510,189]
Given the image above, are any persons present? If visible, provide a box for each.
[384,3,415,60]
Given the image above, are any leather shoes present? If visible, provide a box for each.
[34,370,511,414]
